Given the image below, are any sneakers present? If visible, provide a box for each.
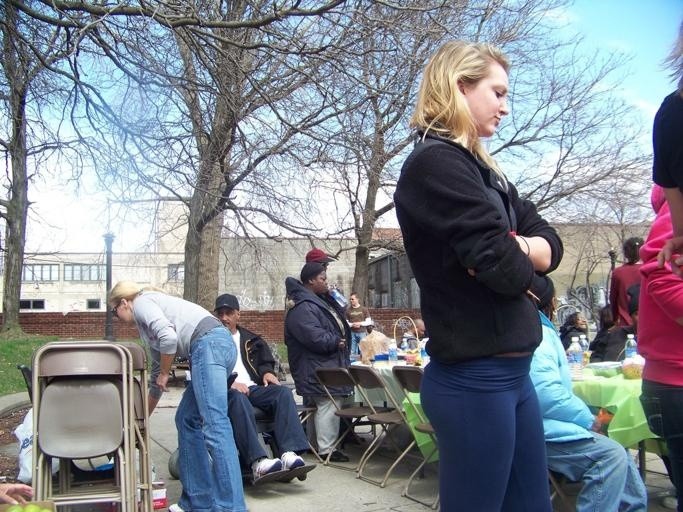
[168,504,186,512]
[663,496,678,508]
[340,433,364,444]
[252,457,282,480]
[281,451,304,469]
[319,452,349,461]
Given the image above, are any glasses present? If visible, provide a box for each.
[110,300,121,319]
[321,262,329,267]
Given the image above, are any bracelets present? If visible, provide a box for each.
[520,235,530,256]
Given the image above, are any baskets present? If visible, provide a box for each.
[394,316,421,359]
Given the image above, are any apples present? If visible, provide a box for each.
[4,503,53,512]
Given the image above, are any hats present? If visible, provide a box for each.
[307,249,334,263]
[528,273,555,309]
[214,294,239,312]
[627,284,640,315]
[301,262,324,283]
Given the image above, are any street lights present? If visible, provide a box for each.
[607,247,616,271]
[101,230,116,340]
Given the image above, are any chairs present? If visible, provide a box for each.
[17,341,152,512]
[296,365,440,509]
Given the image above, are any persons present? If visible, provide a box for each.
[652,23,682,277]
[284,249,426,461]
[526,271,648,512]
[559,237,647,362]
[0,482,34,506]
[637,185,683,512]
[212,294,311,480]
[107,281,249,512]
[392,39,564,512]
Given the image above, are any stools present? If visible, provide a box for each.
[547,466,578,512]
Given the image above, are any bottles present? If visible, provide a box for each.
[388,338,398,369]
[400,338,409,351]
[421,339,431,368]
[328,285,349,308]
[624,334,638,358]
[568,337,582,382]
[580,335,589,353]
